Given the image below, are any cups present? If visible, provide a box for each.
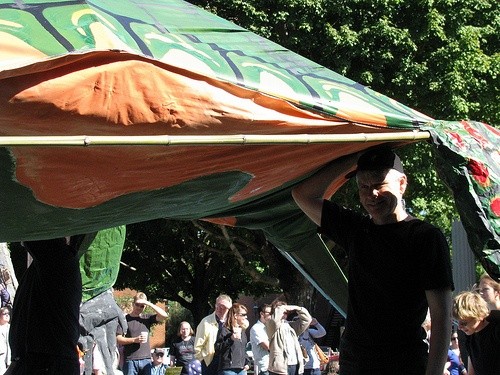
[140,332,148,343]
[234,328,242,339]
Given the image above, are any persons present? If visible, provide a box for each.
[169,321,203,375]
[77,273,500,375]
[0,265,13,375]
[292,143,454,375]
[115,293,169,375]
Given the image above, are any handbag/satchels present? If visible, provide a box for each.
[314,343,329,364]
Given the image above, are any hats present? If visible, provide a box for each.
[345,149,403,178]
[154,348,164,354]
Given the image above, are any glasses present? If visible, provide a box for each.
[0,312,11,315]
[451,337,458,341]
[238,313,247,316]
[460,318,475,326]
